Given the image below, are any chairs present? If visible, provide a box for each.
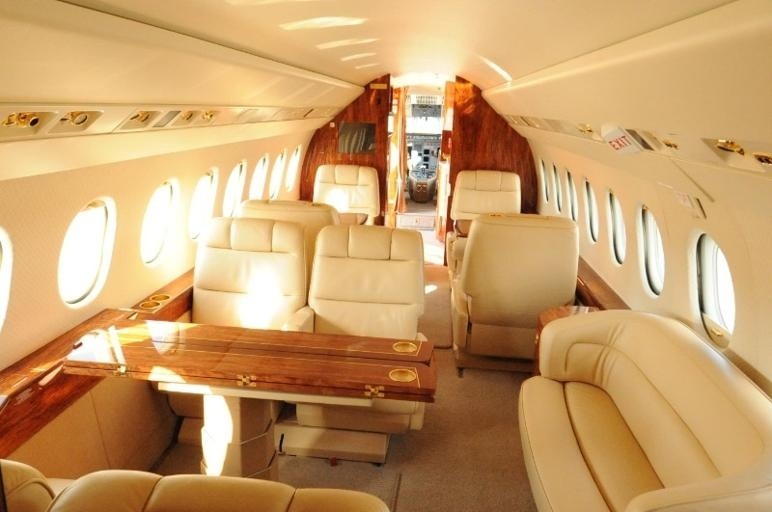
[309,164,384,230]
[447,213,580,375]
[0,455,73,511]
[158,214,310,432]
[445,170,522,288]
[283,223,432,434]
[43,467,389,512]
[239,197,346,286]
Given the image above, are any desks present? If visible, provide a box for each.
[63,319,437,483]
[326,209,369,228]
[455,220,472,237]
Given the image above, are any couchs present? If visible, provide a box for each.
[516,310,772,512]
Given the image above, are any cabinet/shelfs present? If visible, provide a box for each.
[533,305,599,377]
